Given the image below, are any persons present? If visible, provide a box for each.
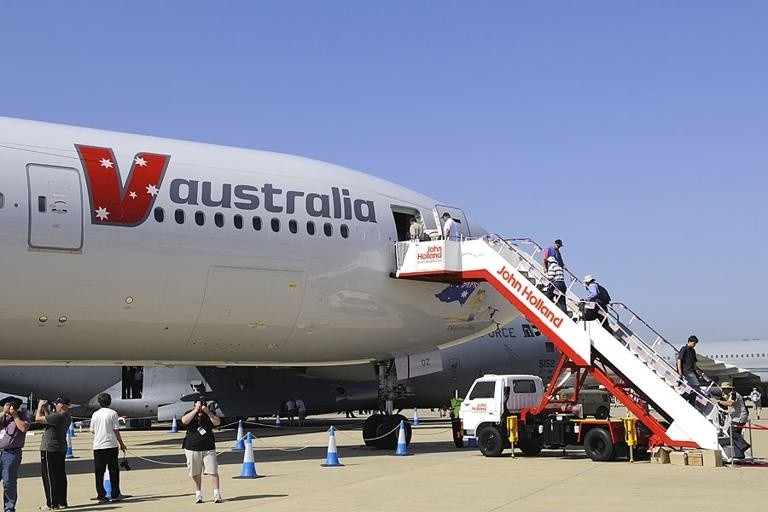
[581,274,612,336]
[90,393,128,503]
[181,399,222,502]
[676,335,700,408]
[122,367,142,399]
[717,382,751,459]
[542,239,566,272]
[408,218,424,241]
[442,213,459,241]
[335,404,371,419]
[750,387,762,420]
[545,255,567,314]
[35,392,71,510]
[0,397,30,512]
[284,397,297,428]
[294,396,307,428]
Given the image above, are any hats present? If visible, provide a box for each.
[721,381,735,389]
[547,257,557,263]
[57,394,71,406]
[443,213,450,217]
[583,275,596,283]
[409,217,416,221]
[555,239,565,247]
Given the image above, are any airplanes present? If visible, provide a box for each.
[650,339,768,406]
[0,312,569,421]
[0,117,523,450]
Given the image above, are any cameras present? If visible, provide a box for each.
[9,400,20,411]
[197,395,209,409]
[35,397,54,407]
[120,460,131,470]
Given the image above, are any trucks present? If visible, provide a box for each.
[550,386,610,420]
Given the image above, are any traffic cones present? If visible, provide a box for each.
[232,430,264,480]
[395,420,413,456]
[274,414,282,429]
[319,425,344,467]
[89,464,131,500]
[411,407,420,426]
[170,415,179,433]
[234,419,248,450]
[68,420,77,437]
[462,438,478,448]
[64,428,80,460]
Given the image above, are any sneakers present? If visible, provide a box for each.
[741,445,752,452]
[99,496,109,503]
[733,455,745,459]
[0,396,22,406]
[214,494,222,503]
[112,494,122,501]
[196,495,203,503]
[39,502,68,510]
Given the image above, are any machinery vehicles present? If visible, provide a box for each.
[393,238,759,468]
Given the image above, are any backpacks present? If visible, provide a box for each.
[752,392,760,401]
[594,281,611,304]
